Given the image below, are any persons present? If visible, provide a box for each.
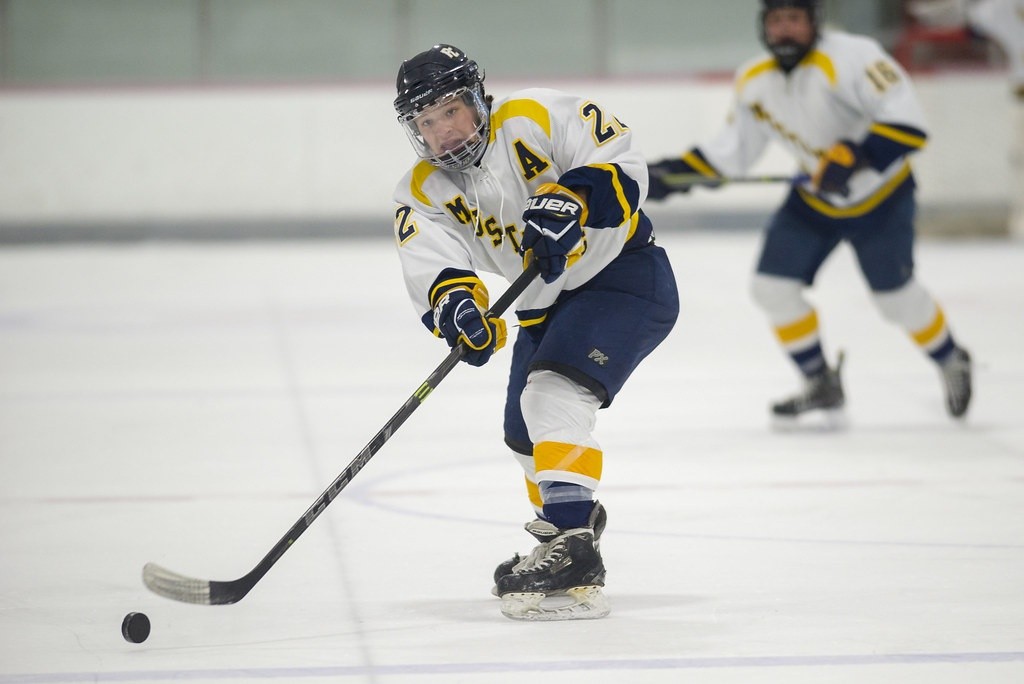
[391,45,680,619]
[647,0,974,434]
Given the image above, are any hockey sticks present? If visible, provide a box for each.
[142,258,550,607]
[652,174,812,185]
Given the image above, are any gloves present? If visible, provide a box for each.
[519,183,588,284]
[432,290,508,367]
[645,159,697,199]
[795,138,864,201]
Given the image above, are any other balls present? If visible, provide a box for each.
[121,612,152,646]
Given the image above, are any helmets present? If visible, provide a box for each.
[394,45,490,166]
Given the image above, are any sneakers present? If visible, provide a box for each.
[942,345,973,415]
[491,500,612,622]
[773,351,848,431]
